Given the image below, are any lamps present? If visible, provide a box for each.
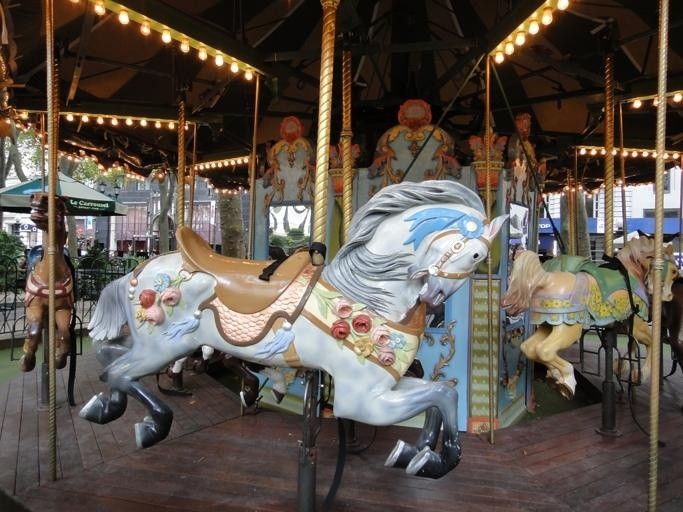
[577,147,681,159]
[3,112,250,172]
[493,0,571,66]
[66,0,253,81]
[631,93,683,111]
